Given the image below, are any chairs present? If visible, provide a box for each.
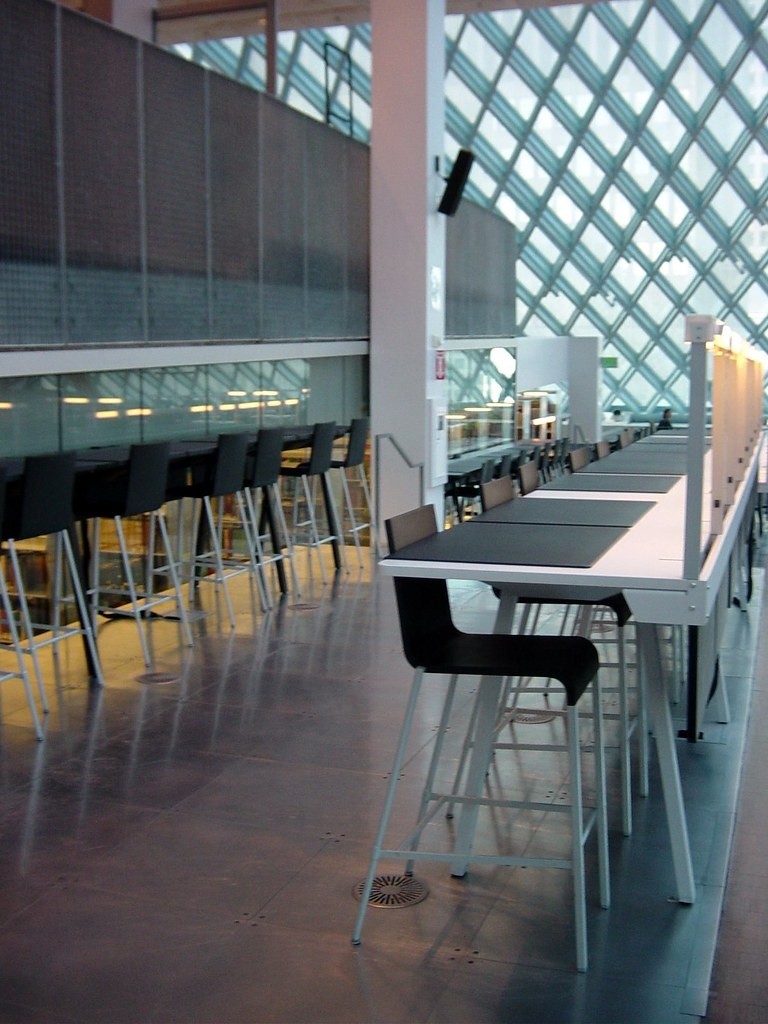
[349,419,657,972]
[0,417,383,741]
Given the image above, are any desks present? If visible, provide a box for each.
[62,439,285,645]
[446,438,560,519]
[0,456,119,677]
[374,425,759,904]
[196,422,362,593]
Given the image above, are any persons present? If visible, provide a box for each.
[657,409,673,429]
